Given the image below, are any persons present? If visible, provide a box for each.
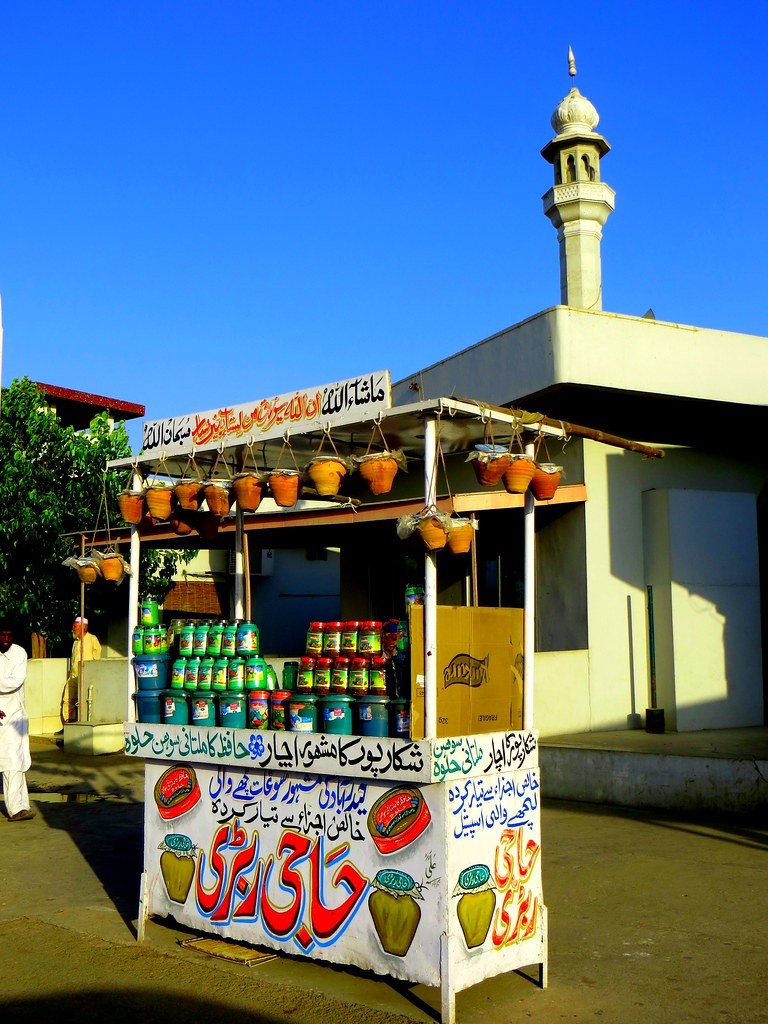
[54,617,101,733]
[0,616,36,821]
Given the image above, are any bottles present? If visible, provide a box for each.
[134,597,298,690]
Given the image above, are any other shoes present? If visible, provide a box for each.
[7,809,34,821]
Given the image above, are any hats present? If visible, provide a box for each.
[75,617,88,624]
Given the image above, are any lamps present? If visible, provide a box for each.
[409,382,420,391]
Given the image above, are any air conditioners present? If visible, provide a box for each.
[230,549,275,577]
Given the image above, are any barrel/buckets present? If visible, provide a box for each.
[131,689,166,723]
[133,654,171,689]
[166,690,409,738]
